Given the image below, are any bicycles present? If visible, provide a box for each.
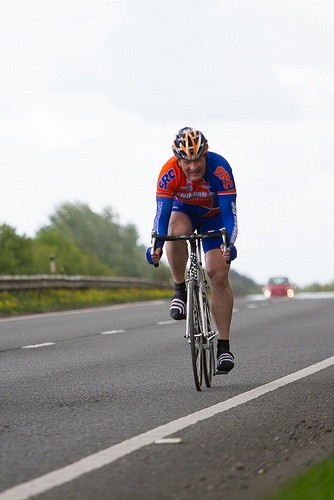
[150,226,228,393]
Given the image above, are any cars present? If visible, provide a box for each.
[264,277,293,298]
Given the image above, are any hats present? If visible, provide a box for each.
[173,128,208,160]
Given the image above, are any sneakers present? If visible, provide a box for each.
[217,347,235,372]
[169,291,188,319]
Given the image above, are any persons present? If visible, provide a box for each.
[145,126,238,371]
